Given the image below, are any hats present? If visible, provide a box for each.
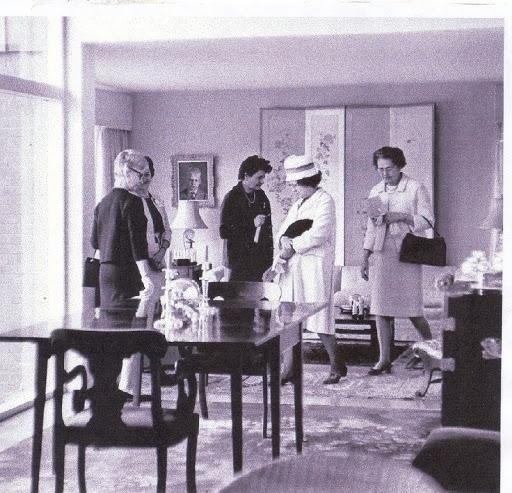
[284,155,318,181]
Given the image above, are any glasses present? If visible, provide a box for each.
[130,167,143,179]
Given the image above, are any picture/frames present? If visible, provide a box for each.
[169,155,215,207]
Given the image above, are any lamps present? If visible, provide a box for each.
[172,201,207,264]
[480,198,503,234]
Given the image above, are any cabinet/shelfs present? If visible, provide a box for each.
[170,264,203,294]
[441,271,500,432]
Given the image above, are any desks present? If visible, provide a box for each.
[0,303,334,493]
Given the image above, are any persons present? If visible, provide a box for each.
[90,149,161,306]
[118,157,172,296]
[219,155,273,282]
[360,146,432,375]
[179,166,208,200]
[268,155,347,385]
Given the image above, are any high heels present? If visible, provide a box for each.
[270,374,298,386]
[369,363,391,375]
[324,367,347,383]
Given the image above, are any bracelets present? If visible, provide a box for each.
[159,247,166,251]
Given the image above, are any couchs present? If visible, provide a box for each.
[203,265,454,348]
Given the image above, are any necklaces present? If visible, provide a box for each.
[244,191,256,208]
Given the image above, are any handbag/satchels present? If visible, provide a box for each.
[83,249,99,287]
[399,216,446,266]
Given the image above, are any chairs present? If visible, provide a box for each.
[208,448,443,493]
[47,330,199,492]
[412,425,500,493]
[198,282,283,422]
